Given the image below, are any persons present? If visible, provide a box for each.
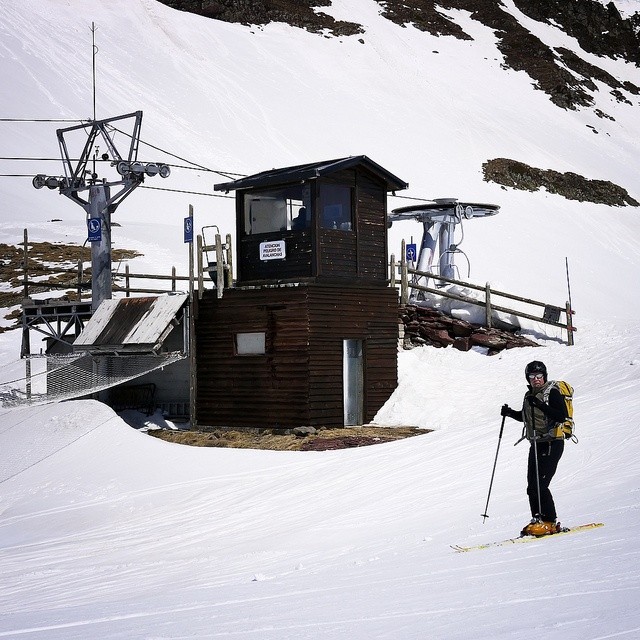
[501,361,572,536]
[292,207,307,229]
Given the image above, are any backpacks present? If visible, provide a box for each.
[543,381,575,441]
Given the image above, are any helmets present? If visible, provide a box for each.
[525,360,547,383]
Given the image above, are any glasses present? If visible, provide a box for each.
[525,373,543,378]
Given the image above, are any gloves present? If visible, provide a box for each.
[529,395,543,408]
[501,404,511,415]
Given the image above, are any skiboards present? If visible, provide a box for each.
[450,523,602,551]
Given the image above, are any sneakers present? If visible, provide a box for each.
[524,520,556,536]
